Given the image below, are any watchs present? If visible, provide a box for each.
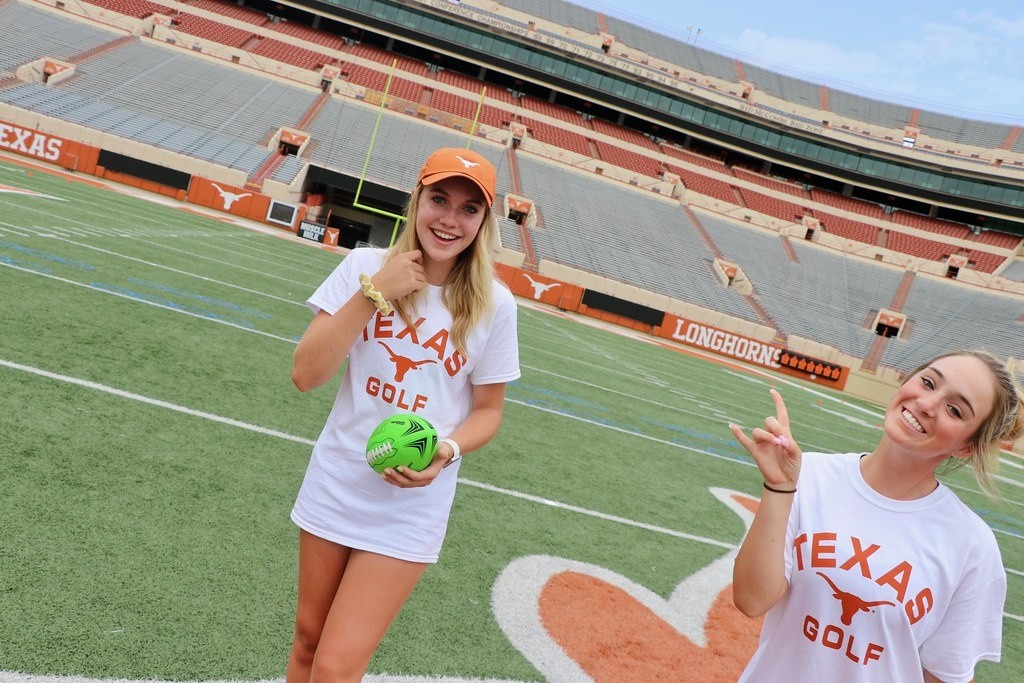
[440,439,459,470]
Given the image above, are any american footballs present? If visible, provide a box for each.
[365,414,439,475]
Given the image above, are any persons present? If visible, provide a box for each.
[287,147,522,683]
[727,350,1024,683]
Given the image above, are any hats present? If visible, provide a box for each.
[417,148,496,210]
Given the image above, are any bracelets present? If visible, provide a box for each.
[764,482,797,493]
[359,274,389,317]
